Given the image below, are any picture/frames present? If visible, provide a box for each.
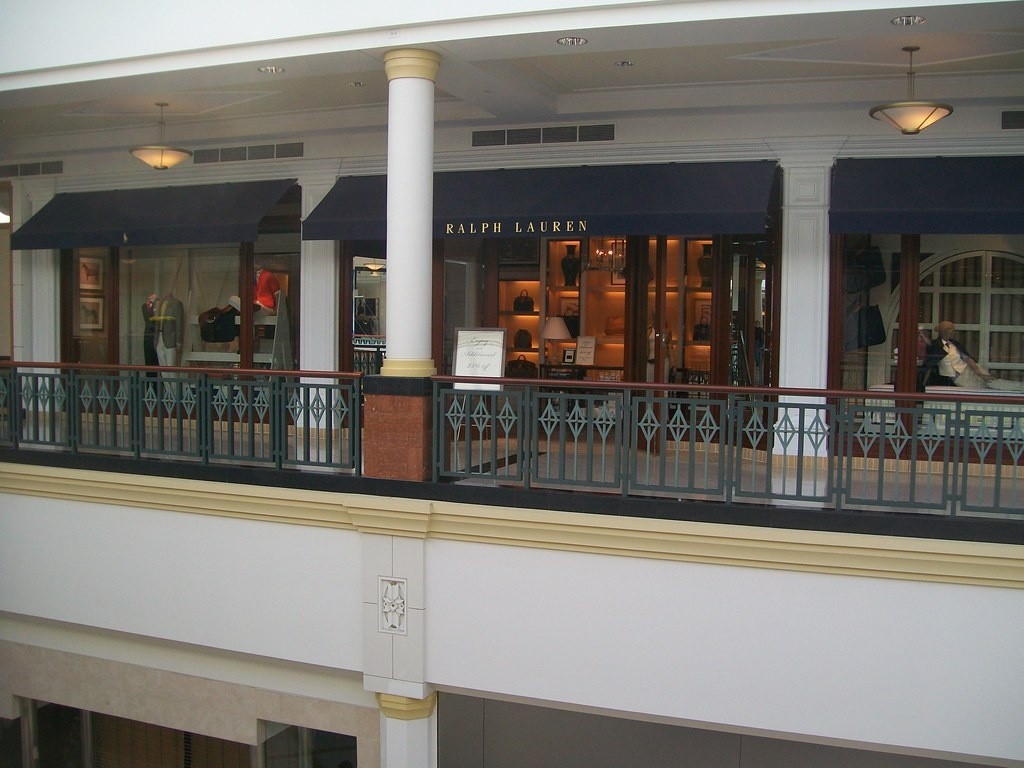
[611,242,626,286]
[497,237,540,267]
[80,294,105,332]
[691,297,712,339]
[79,254,104,294]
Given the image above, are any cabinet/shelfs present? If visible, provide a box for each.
[685,287,713,346]
[179,287,294,402]
[588,286,624,344]
[497,270,540,353]
[545,237,582,342]
[648,287,678,346]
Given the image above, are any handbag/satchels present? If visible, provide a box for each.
[606,316,625,334]
[845,245,886,294]
[515,329,532,348]
[514,288,533,311]
[507,355,536,379]
[692,316,712,340]
[841,306,886,351]
[557,308,580,338]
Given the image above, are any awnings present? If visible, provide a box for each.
[9,178,296,250]
[302,161,782,239]
[828,156,1024,235]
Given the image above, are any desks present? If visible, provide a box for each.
[539,364,689,429]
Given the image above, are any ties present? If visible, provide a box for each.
[945,343,950,347]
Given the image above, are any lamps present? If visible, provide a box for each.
[539,314,572,365]
[868,44,954,135]
[129,102,192,170]
[364,258,385,270]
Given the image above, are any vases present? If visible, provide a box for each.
[561,245,579,287]
[697,243,712,287]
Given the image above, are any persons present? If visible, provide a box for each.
[754,321,763,367]
[647,308,676,421]
[143,291,184,398]
[254,264,280,315]
[919,321,1024,390]
